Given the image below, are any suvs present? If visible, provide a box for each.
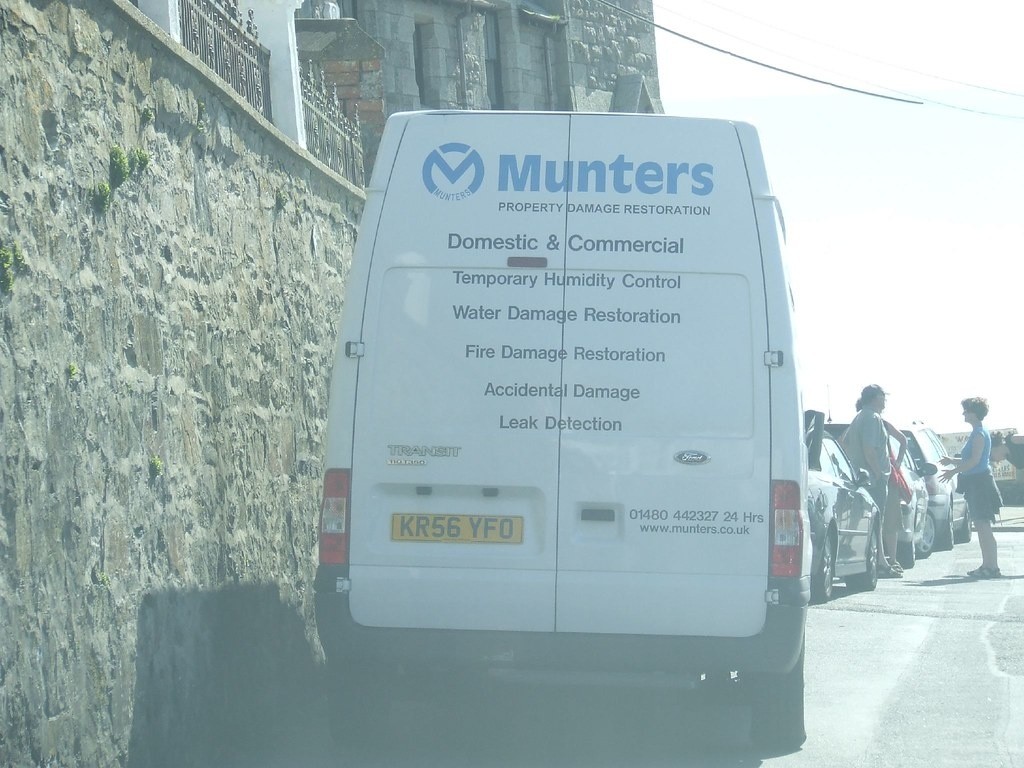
[825,423,940,569]
[886,420,973,568]
[805,425,883,600]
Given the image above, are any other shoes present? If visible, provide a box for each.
[889,560,904,573]
[876,564,902,579]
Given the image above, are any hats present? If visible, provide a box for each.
[861,385,889,404]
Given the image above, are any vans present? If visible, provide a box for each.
[309,107,826,745]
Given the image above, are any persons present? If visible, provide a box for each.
[937,397,1004,575]
[837,384,911,578]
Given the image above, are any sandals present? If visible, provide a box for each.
[967,566,1002,578]
